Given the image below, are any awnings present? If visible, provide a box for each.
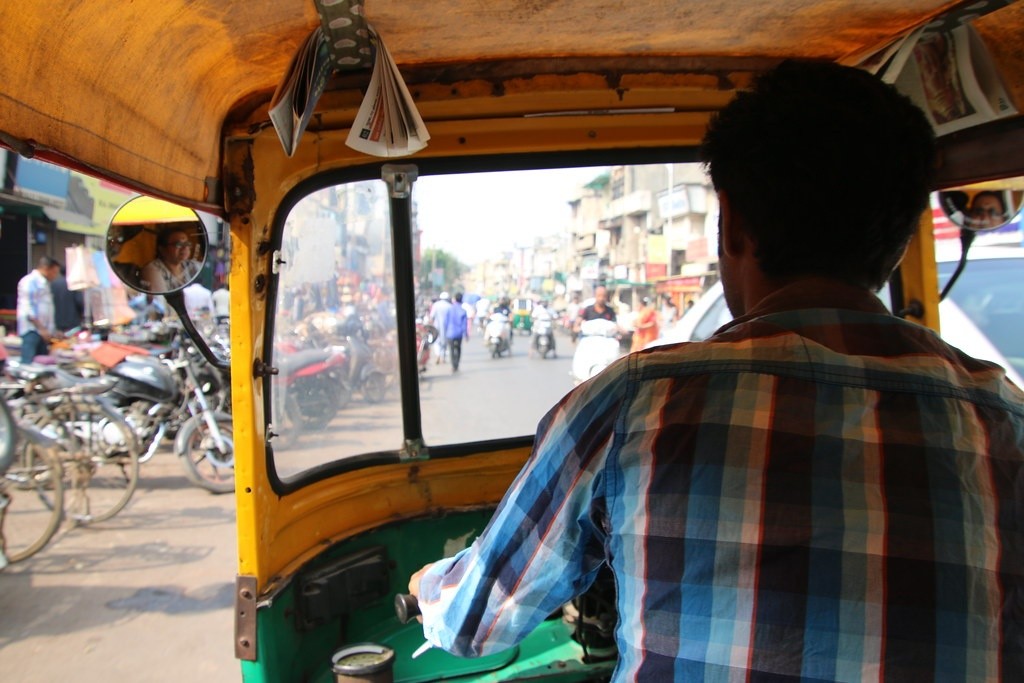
[33,204,105,237]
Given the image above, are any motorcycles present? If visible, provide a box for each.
[511,298,535,335]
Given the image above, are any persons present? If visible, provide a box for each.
[462,295,514,357]
[416,290,452,365]
[408,55,1024,683]
[129,276,230,325]
[968,191,1006,230]
[16,256,61,363]
[51,266,84,333]
[573,283,622,340]
[142,229,202,293]
[567,297,580,342]
[532,299,557,359]
[443,293,469,372]
[630,294,695,353]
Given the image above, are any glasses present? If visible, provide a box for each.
[164,241,192,248]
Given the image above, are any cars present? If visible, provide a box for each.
[640,243,1024,392]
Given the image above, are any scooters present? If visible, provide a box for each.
[481,313,513,359]
[569,317,626,388]
[529,314,560,359]
[0,296,438,573]
